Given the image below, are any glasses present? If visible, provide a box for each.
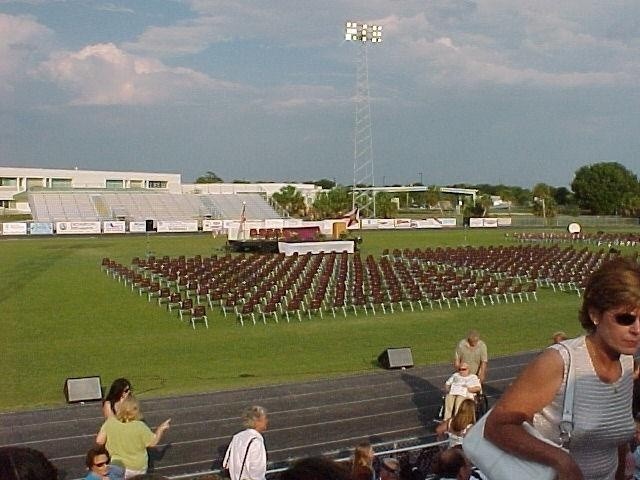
[458,368,467,371]
[92,457,111,467]
[124,388,129,393]
[604,308,640,326]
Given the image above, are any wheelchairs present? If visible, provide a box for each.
[435,393,488,425]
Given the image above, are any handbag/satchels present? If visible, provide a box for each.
[462,407,570,479]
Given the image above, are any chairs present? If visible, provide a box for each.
[379,227,638,301]
[102,248,541,331]
[248,228,283,241]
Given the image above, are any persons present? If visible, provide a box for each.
[222,405,268,480]
[84,378,171,480]
[275,443,487,480]
[1,447,57,480]
[483,256,640,479]
[624,357,640,479]
[436,331,488,449]
[554,332,568,344]
